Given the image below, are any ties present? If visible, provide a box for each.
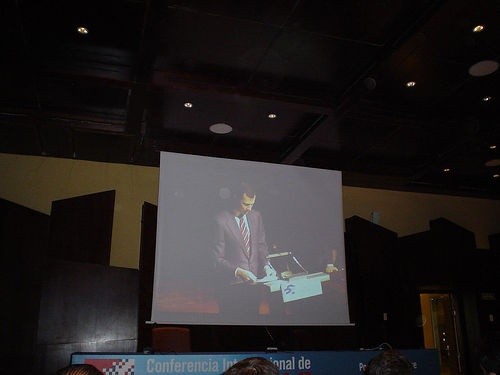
[240,217,250,257]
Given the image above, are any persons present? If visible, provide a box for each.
[211,183,270,315]
[221,357,279,375]
[56,364,103,375]
[364,350,416,375]
[480,351,500,375]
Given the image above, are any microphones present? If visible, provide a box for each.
[266,252,291,258]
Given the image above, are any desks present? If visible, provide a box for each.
[69,347,440,375]
[218,273,326,322]
